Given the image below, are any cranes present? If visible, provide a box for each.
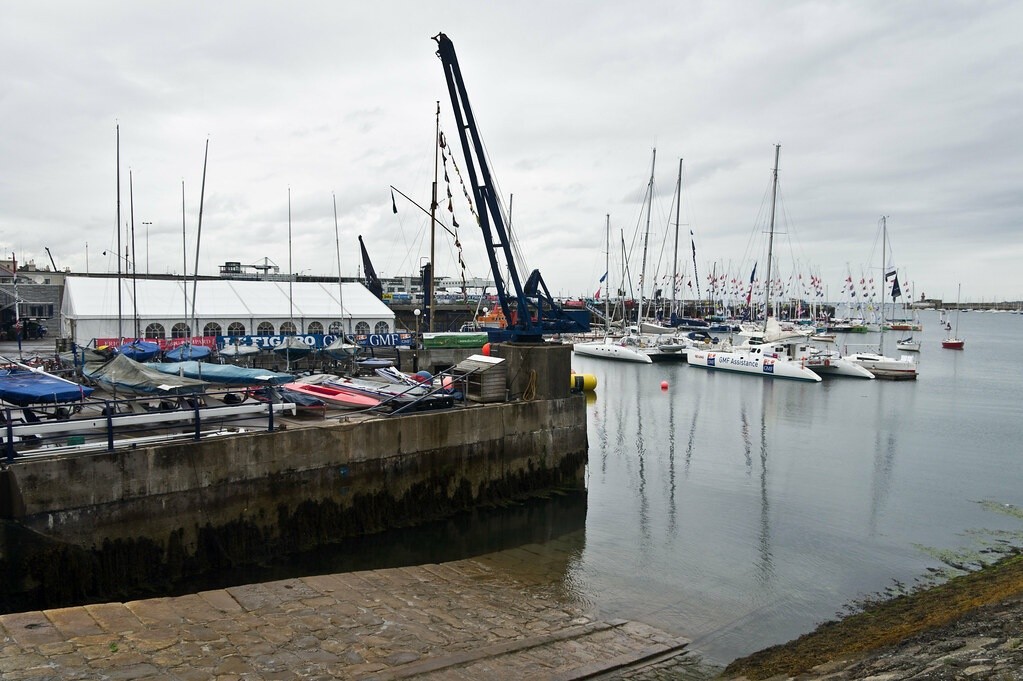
[219,256,278,281]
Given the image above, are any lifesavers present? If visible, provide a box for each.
[824,359,830,368]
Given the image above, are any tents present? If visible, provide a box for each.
[0,334,362,422]
[62,276,396,348]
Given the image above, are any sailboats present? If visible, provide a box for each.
[569,142,920,383]
[962,296,1023,314]
[940,312,947,325]
[472,193,589,336]
[944,312,951,330]
[0,117,455,422]
[940,281,966,350]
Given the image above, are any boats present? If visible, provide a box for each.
[570,370,597,391]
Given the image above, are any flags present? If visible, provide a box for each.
[745,287,752,304]
[750,262,757,283]
[884,255,897,294]
[891,274,901,302]
[599,271,608,283]
[595,289,600,299]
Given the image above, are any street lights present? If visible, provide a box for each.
[300,269,311,281]
[142,221,152,279]
[379,272,383,278]
[419,256,428,276]
